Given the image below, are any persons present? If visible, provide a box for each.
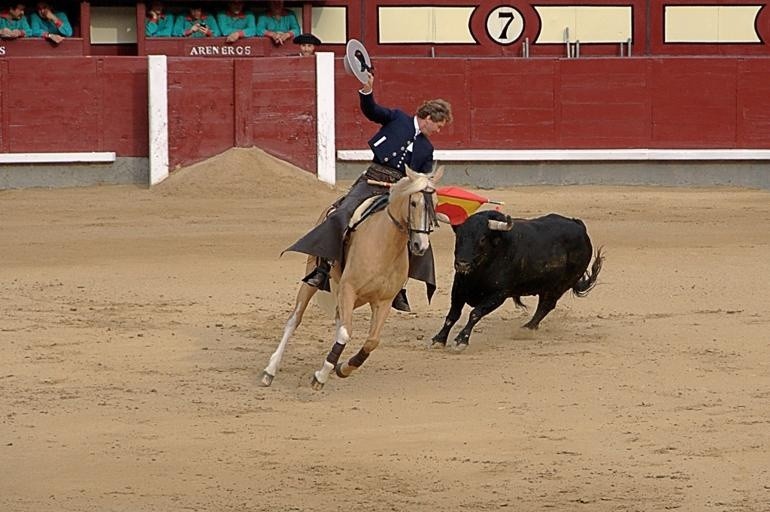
[1,0,32,40]
[172,0,221,38]
[293,33,321,57]
[216,1,256,44]
[144,0,175,38]
[279,71,454,313]
[257,1,300,47]
[29,1,73,44]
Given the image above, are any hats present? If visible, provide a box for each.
[293,33,320,45]
[344,39,371,85]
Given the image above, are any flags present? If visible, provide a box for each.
[431,186,489,228]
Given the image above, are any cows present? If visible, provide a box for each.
[428,210,608,351]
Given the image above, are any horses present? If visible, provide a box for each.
[260,163,446,391]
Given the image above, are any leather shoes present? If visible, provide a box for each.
[307,262,329,286]
[393,292,410,310]
